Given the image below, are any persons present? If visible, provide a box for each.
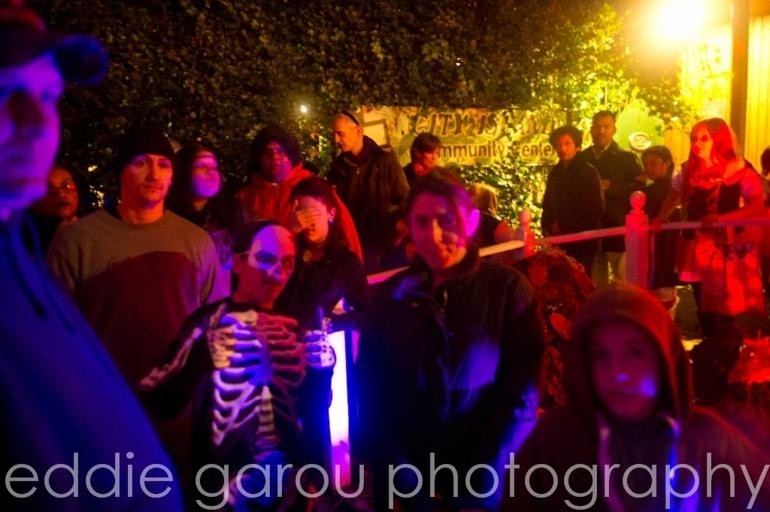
[133,218,337,512]
[28,160,92,254]
[33,129,218,465]
[356,168,547,511]
[541,124,607,279]
[401,131,450,190]
[649,120,770,403]
[232,124,365,275]
[323,110,411,273]
[467,178,531,246]
[0,7,187,512]
[164,138,251,309]
[640,146,690,293]
[230,175,372,511]
[166,128,191,211]
[500,279,768,512]
[581,110,646,286]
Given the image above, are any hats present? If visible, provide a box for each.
[0,7,108,87]
[114,127,175,172]
